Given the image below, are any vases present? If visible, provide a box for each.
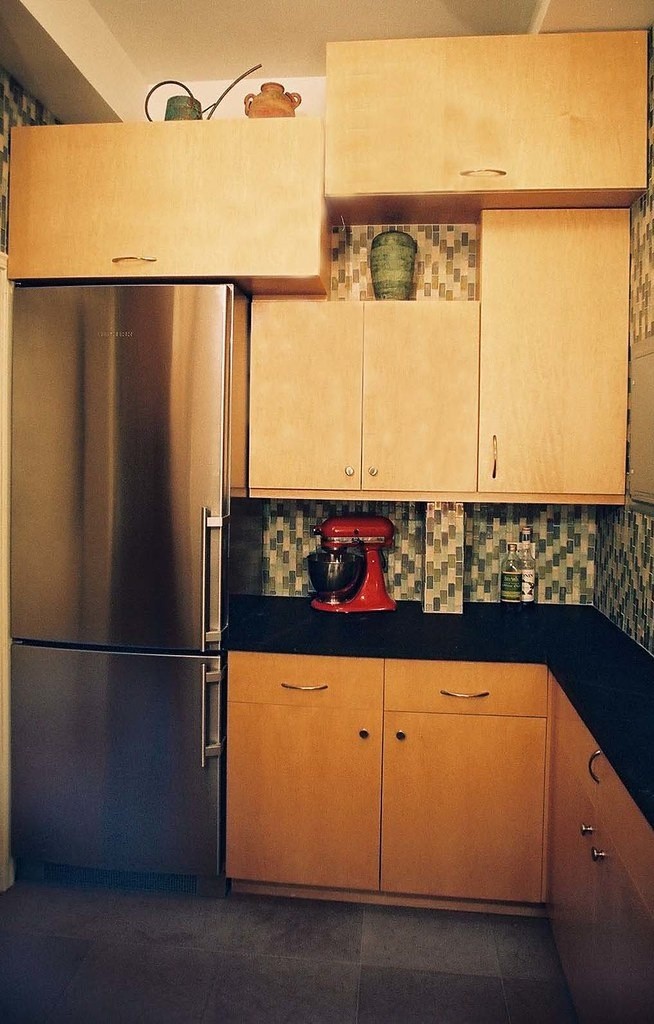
[243,82,302,117]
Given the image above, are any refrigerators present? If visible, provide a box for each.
[6,282,250,902]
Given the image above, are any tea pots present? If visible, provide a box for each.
[144,65,262,121]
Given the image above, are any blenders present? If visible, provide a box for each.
[306,512,397,613]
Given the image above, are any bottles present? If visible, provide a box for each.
[518,527,537,604]
[244,80,301,119]
[500,543,522,605]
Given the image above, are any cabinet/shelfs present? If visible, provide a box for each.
[324,29,650,223]
[224,650,653,1024]
[477,207,631,506]
[6,119,332,296]
[248,297,480,504]
